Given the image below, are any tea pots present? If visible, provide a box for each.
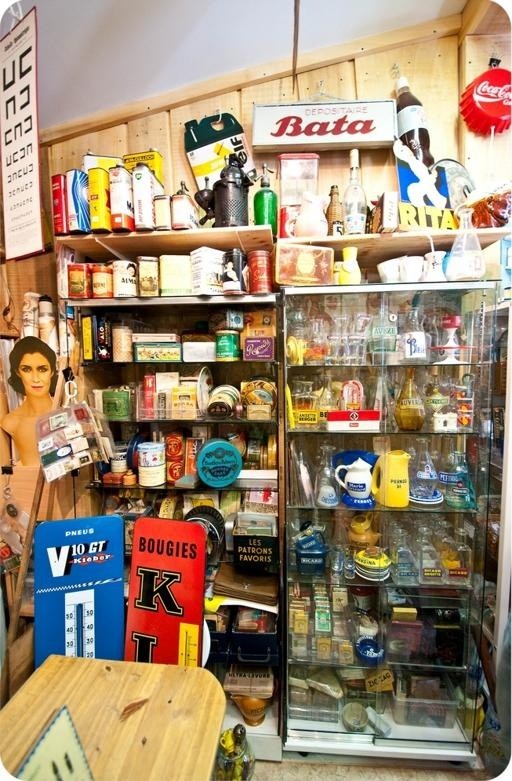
[229,693,273,727]
[283,191,329,243]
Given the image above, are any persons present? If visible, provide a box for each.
[124,262,136,277]
[222,259,239,282]
[3,334,60,469]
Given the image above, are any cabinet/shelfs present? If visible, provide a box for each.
[56,225,511,771]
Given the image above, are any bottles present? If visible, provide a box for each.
[339,245,362,286]
[396,74,436,169]
[213,153,277,241]
[212,730,255,780]
[287,304,476,589]
[443,208,487,282]
[326,149,367,236]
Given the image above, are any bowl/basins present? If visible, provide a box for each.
[340,702,369,733]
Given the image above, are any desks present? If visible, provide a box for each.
[1,653,227,779]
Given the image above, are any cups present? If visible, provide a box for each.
[377,250,447,282]
[110,452,128,474]
